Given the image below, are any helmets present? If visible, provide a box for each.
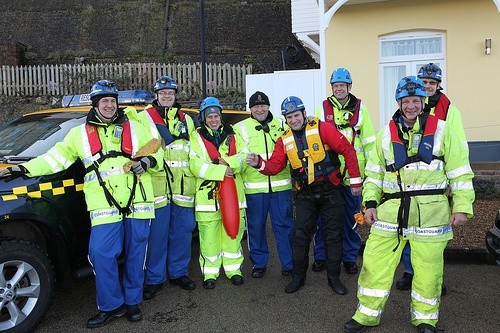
[417,63,441,82]
[330,68,352,84]
[154,76,178,94]
[395,76,426,100]
[281,96,305,116]
[90,80,119,100]
[200,97,223,114]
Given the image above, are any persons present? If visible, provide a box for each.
[312,68,376,274]
[0,80,164,328]
[343,76,473,333]
[246,96,363,295]
[396,63,469,296]
[137,75,196,296]
[233,91,292,278]
[189,96,250,289]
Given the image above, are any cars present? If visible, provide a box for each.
[1,106,269,333]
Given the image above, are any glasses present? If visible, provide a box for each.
[158,92,176,96]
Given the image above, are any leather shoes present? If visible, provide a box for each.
[86,304,126,328]
[231,275,243,285]
[203,279,216,290]
[253,267,265,278]
[312,261,327,271]
[344,320,379,333]
[417,324,436,333]
[284,280,305,293]
[328,281,346,295]
[175,276,196,290]
[397,273,412,290]
[344,262,357,274]
[125,305,142,321]
[143,284,162,299]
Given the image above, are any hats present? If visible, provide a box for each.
[249,91,270,109]
[204,107,220,117]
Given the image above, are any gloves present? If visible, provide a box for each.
[130,157,157,175]
[0,166,27,178]
